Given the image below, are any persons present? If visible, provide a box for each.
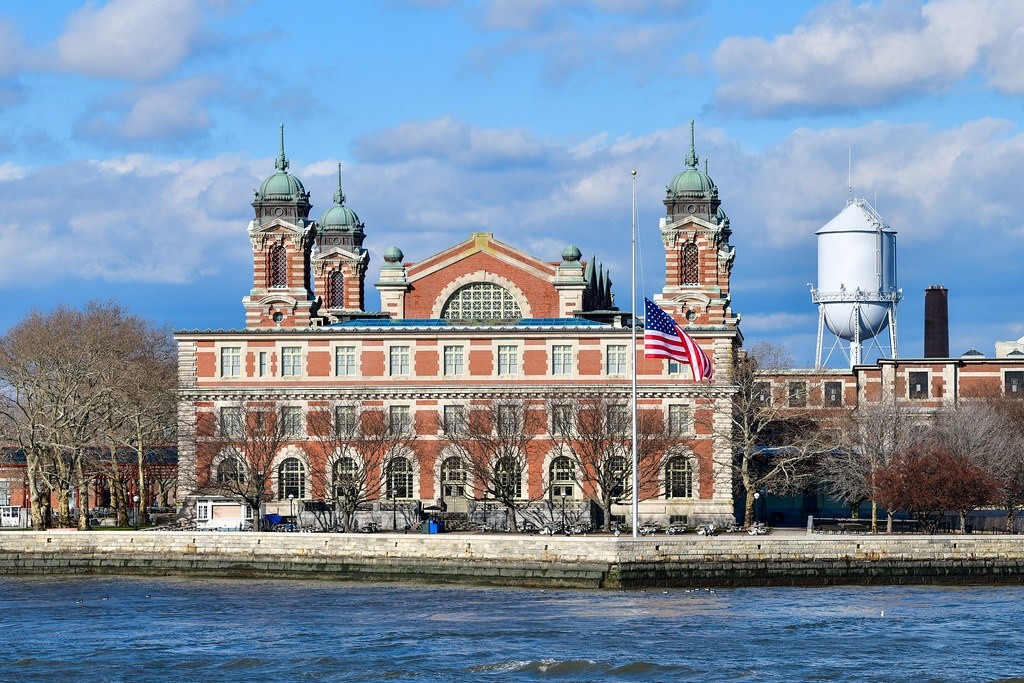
[564,525,572,537]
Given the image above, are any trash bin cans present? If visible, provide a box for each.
[421,519,443,533]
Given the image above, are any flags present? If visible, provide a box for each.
[643,297,712,382]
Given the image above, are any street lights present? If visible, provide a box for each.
[133,496,140,531]
[561,492,567,535]
[392,489,398,531]
[754,493,760,525]
[288,494,294,525]
[26,495,29,529]
[482,488,489,522]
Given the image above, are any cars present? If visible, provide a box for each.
[477,522,771,535]
